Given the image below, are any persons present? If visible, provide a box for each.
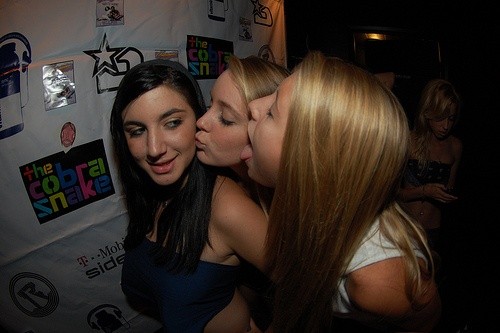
[240,52,441,329]
[375,72,463,236]
[195,55,294,187]
[111,59,269,333]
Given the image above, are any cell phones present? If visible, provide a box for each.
[443,188,453,194]
